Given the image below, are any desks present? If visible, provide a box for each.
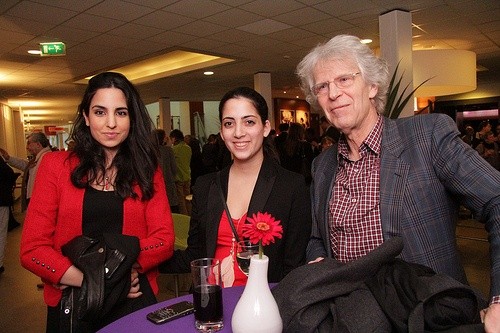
[99,282,279,333]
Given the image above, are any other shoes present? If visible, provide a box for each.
[0,266,5,273]
[37,284,44,289]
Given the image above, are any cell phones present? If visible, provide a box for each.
[146,301,193,324]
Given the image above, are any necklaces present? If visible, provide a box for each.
[106,178,108,190]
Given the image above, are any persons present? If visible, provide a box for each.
[184,135,202,188]
[267,116,340,187]
[460,121,500,171]
[21,72,175,333]
[202,132,230,173]
[0,132,52,288]
[170,129,192,215]
[295,34,500,333]
[158,87,310,289]
[157,133,178,212]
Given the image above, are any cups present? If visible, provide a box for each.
[236,240,261,277]
[190,258,223,333]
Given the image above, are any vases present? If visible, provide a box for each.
[232,255,283,333]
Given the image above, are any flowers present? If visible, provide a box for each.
[241,210,283,259]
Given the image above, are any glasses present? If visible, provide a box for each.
[310,70,362,97]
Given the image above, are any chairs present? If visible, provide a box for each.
[161,212,190,299]
